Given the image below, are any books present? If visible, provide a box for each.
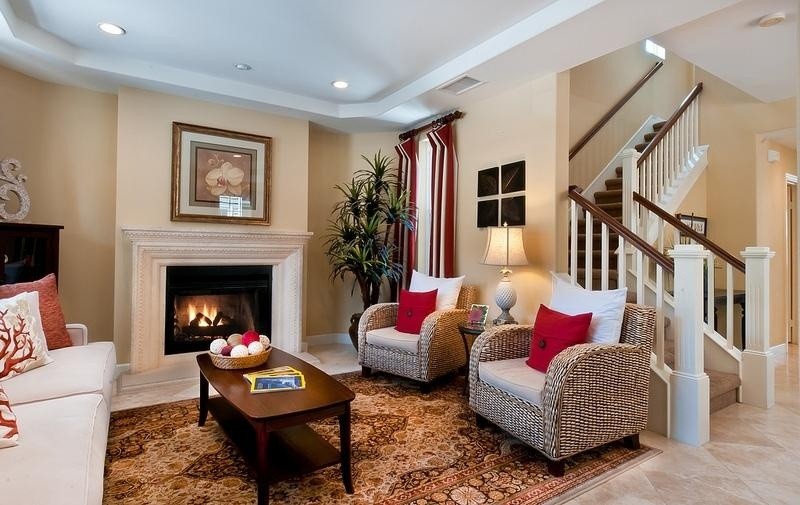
[243,366,306,393]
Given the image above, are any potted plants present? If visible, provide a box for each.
[323,149,420,352]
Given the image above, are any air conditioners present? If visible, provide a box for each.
[480,222,531,325]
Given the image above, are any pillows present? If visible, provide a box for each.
[524,303,593,374]
[0,383,23,449]
[392,286,438,335]
[406,270,466,312]
[0,272,73,350]
[0,291,55,382]
[544,269,627,348]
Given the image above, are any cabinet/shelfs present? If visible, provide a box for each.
[0,221,63,294]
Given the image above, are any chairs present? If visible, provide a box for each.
[465,303,657,478]
[357,268,478,395]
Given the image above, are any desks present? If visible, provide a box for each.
[667,288,746,351]
[452,320,488,398]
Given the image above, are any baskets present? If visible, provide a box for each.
[206,344,273,370]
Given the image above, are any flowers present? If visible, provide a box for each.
[204,151,247,200]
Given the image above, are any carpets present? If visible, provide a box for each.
[99,370,663,505]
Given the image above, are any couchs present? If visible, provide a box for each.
[0,322,116,504]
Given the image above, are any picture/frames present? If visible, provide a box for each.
[171,121,273,226]
[678,214,707,240]
